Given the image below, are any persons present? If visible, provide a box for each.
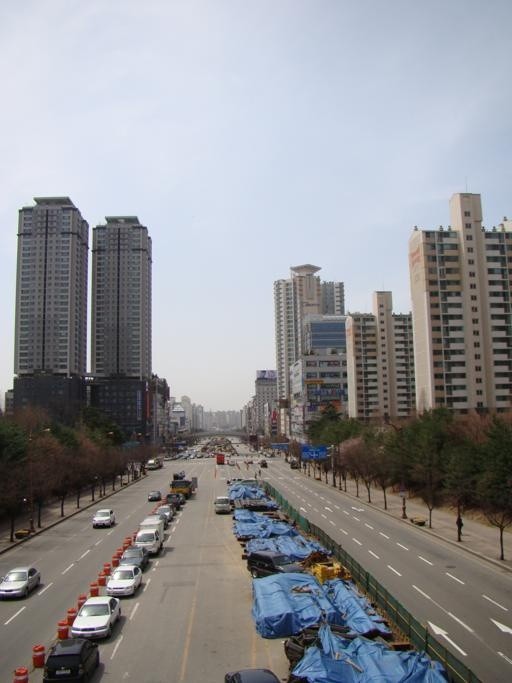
[455,516,464,535]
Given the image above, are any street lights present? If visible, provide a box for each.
[98,429,152,496]
[30,427,53,533]
[382,420,410,519]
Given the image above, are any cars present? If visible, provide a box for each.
[222,667,281,683]
[286,456,297,462]
[41,469,199,682]
[243,444,276,467]
[179,435,241,465]
[290,459,298,469]
[0,564,42,601]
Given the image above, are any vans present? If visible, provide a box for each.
[212,494,232,515]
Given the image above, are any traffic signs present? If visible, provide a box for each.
[272,443,288,449]
[301,443,327,460]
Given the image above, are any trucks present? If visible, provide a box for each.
[147,454,164,469]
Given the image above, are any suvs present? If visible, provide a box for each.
[246,546,306,579]
